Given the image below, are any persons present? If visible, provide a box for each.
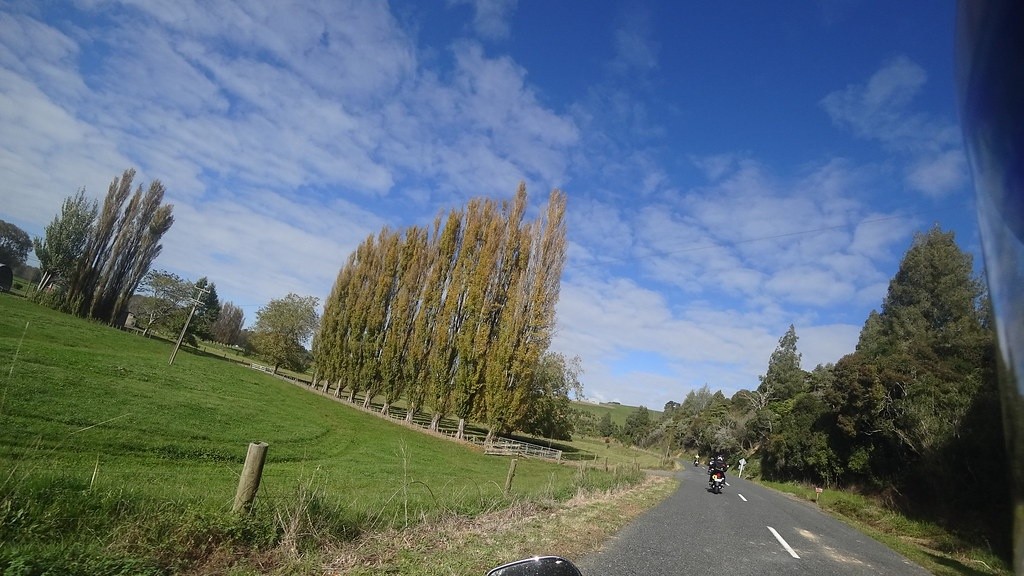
[708,455,727,483]
[694,454,700,464]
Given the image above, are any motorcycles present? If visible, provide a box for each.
[709,462,729,494]
[694,458,699,466]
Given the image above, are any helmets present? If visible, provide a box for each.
[717,455,724,461]
